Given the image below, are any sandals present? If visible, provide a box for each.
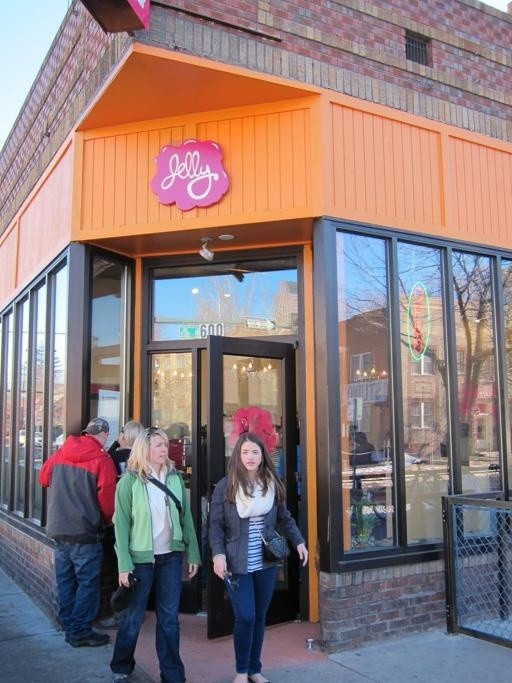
[248,672,272,683]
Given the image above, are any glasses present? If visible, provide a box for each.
[223,570,241,593]
[120,426,124,433]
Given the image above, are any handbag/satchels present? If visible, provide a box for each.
[263,536,291,564]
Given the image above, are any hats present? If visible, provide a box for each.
[81,418,109,435]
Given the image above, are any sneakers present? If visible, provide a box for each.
[65,631,110,647]
[96,616,121,629]
[112,672,128,683]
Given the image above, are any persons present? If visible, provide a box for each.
[209,434,308,683]
[38,417,117,647]
[94,421,201,683]
[349,432,378,465]
